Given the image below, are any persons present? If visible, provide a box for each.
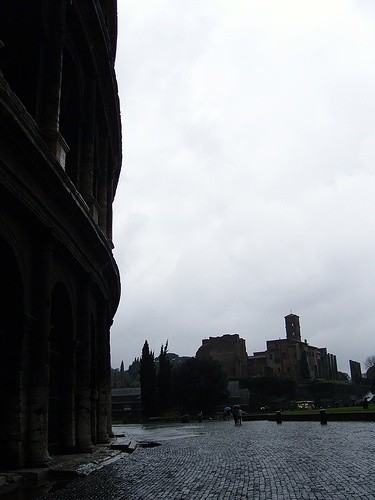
[233,406,239,425]
[237,407,242,424]
[226,409,230,420]
[259,400,365,414]
